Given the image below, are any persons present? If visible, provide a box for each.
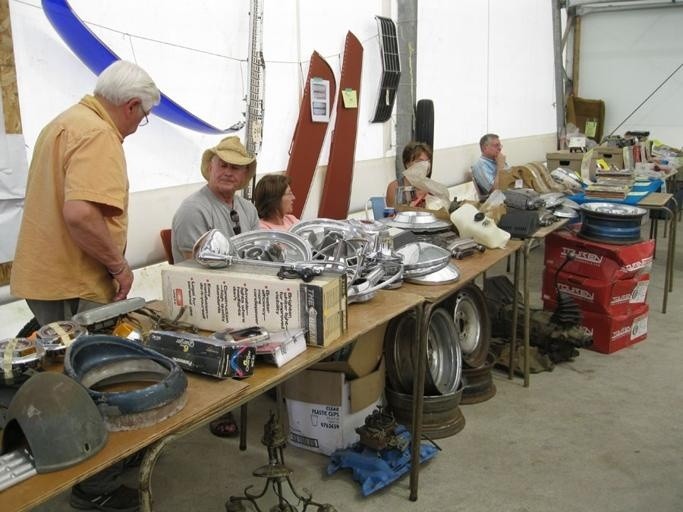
[7,59,162,334]
[249,170,303,235]
[468,132,513,197]
[169,135,263,268]
[382,139,436,210]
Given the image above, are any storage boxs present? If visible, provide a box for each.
[545,146,624,177]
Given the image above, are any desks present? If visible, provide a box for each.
[0,164,678,512]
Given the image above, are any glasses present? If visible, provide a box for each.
[230,210,241,235]
[139,104,149,127]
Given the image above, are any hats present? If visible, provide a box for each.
[201,136,257,189]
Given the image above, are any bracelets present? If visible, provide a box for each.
[102,259,128,277]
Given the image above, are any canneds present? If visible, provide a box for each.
[383,206,397,218]
